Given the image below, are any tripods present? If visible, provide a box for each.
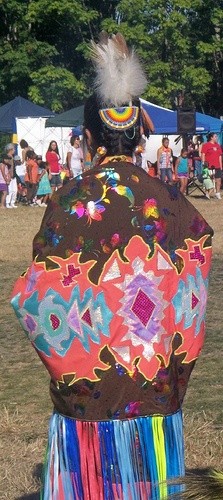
[174,135,208,196]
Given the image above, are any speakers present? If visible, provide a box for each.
[177,107,196,135]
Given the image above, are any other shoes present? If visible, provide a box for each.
[6,202,18,209]
[205,194,211,199]
[35,199,47,208]
[214,193,222,200]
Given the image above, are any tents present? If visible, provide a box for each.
[0,96,223,173]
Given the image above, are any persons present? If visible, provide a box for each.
[7,29,214,500]
[0,135,93,208]
[132,132,223,201]
